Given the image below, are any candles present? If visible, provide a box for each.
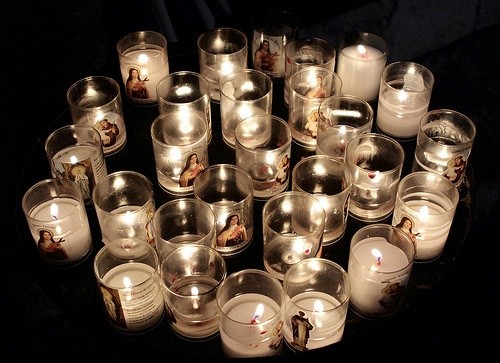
[337,44,384,101]
[349,241,409,311]
[223,302,278,356]
[378,89,428,137]
[121,50,169,100]
[33,202,90,260]
[284,297,343,349]
[393,204,451,259]
[107,270,164,330]
[172,286,220,338]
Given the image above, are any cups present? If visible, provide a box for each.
[22,178,93,268]
[151,10,476,358]
[94,237,165,336]
[44,124,108,208]
[92,170,156,263]
[66,75,127,158]
[116,30,170,108]
